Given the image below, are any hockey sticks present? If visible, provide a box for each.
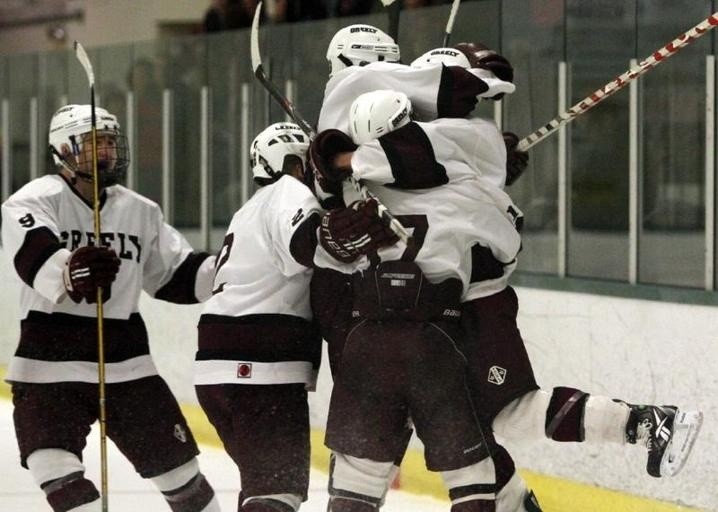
[250,2,419,249]
[73,39,107,512]
[442,0,460,47]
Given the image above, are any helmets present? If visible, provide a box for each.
[48,104,130,187]
[248,122,311,187]
[325,24,471,149]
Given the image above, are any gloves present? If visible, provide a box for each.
[62,246,121,305]
[319,197,399,262]
[306,130,354,210]
[474,49,514,100]
[504,131,529,185]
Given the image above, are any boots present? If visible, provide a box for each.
[613,399,677,476]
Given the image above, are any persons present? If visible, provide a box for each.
[0,95,219,512]
[96,0,387,228]
[193,24,704,512]
